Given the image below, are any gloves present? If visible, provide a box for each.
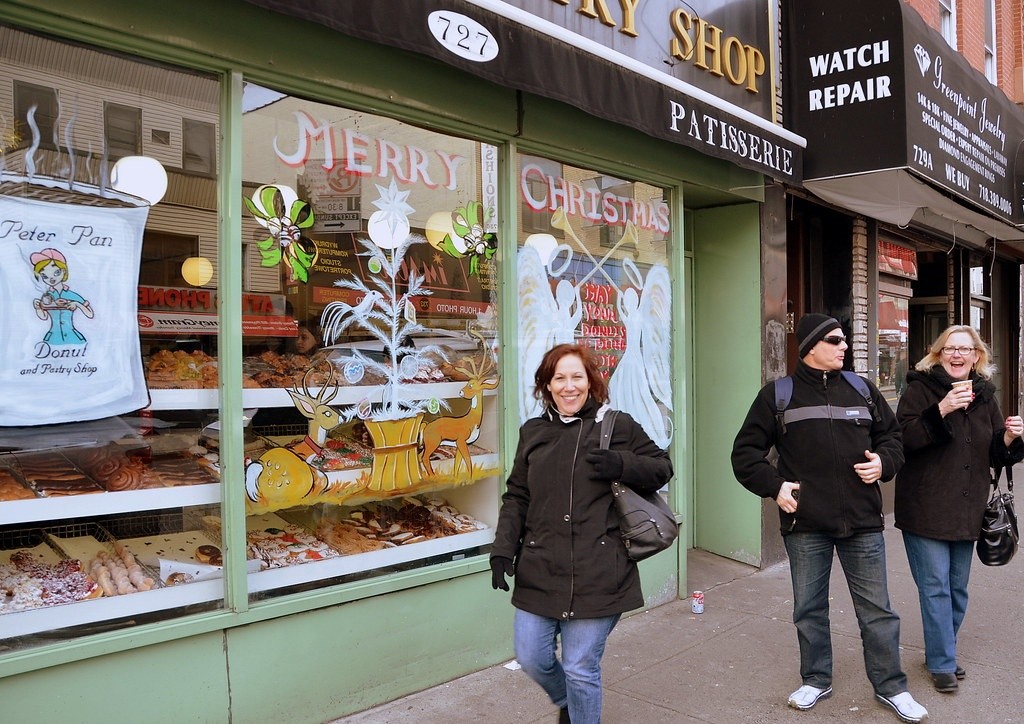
[490,556,514,592]
[586,448,623,482]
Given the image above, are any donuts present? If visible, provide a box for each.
[166,572,195,587]
[195,495,489,572]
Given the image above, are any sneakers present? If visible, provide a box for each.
[787,683,833,711]
[874,690,930,724]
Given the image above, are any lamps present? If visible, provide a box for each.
[426,187,468,252]
[182,256,213,286]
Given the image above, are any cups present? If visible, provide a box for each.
[951,379,972,394]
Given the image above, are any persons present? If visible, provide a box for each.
[730,313,928,724]
[246,299,336,439]
[893,325,1023,692]
[361,336,417,446]
[490,345,675,723]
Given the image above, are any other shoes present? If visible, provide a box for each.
[925,658,966,678]
[932,672,958,692]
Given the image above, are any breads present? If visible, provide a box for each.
[0,440,220,502]
[0,540,154,616]
[142,344,498,390]
[244,422,488,472]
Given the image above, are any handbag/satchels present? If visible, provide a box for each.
[599,408,678,562]
[976,429,1019,566]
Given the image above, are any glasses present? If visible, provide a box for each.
[940,346,978,355]
[821,335,846,345]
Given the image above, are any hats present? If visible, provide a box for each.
[795,312,843,359]
